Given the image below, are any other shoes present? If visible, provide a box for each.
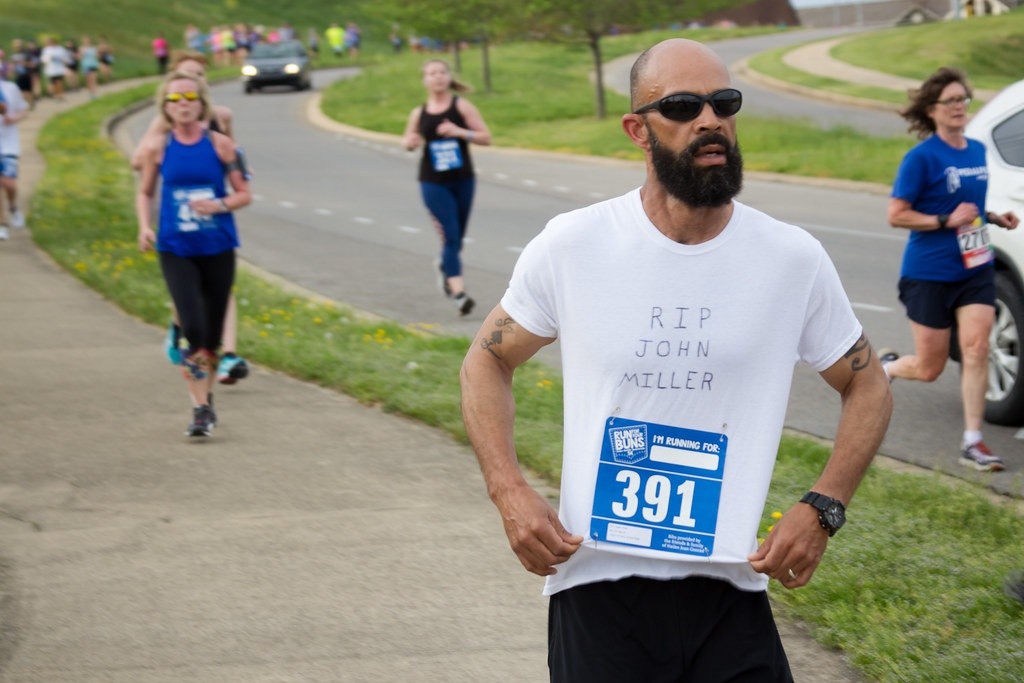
[0,206,27,240]
[454,292,474,316]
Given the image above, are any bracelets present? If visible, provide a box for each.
[213,198,229,213]
[466,129,474,143]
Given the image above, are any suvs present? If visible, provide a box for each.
[949,77,1024,428]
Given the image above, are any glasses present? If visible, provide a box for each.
[632,89,742,122]
[163,91,201,102]
[935,96,972,107]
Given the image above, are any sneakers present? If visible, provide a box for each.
[167,320,191,365]
[958,440,1006,472]
[876,347,900,384]
[183,403,216,436]
[216,351,247,386]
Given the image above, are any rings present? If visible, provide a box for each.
[789,568,796,579]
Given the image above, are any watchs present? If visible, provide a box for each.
[799,491,846,537]
[937,214,948,230]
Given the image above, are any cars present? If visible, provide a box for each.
[243,43,310,92]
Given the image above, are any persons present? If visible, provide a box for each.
[459,37,894,683]
[131,50,253,437]
[153,30,171,76]
[0,34,116,112]
[403,59,491,316]
[0,81,27,241]
[186,21,483,67]
[874,68,1019,471]
[608,15,788,36]
